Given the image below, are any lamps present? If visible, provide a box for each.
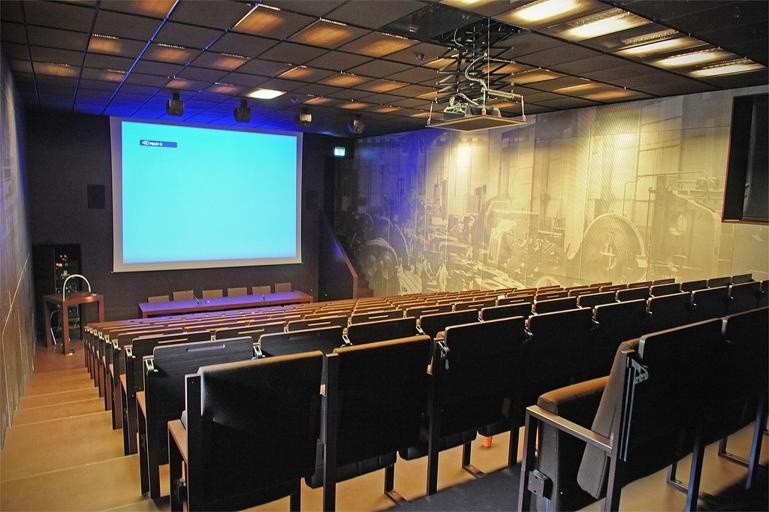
[166,91,185,116]
[300,107,312,127]
[234,99,251,121]
[352,113,365,134]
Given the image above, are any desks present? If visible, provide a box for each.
[42,291,104,357]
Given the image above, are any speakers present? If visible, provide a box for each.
[721,92,769,224]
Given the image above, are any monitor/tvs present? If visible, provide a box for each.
[110,116,304,275]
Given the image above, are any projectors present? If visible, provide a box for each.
[425,88,527,132]
